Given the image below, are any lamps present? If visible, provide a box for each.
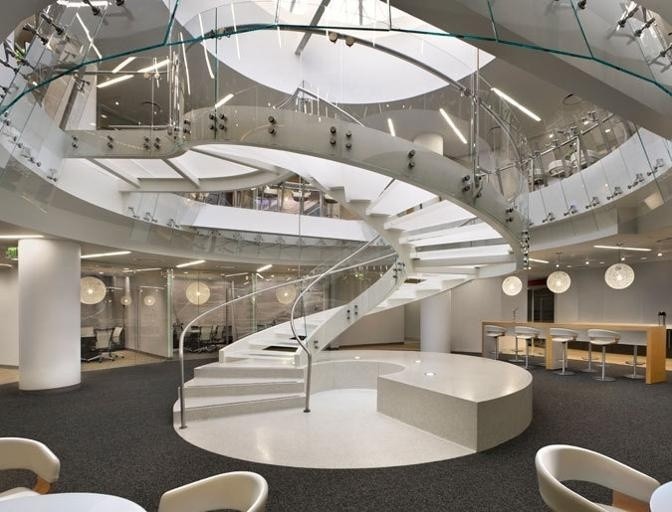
[500,241,635,295]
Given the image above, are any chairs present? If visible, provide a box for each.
[526,148,602,191]
[0,435,63,501]
[482,321,647,385]
[80,326,126,365]
[533,441,661,512]
[153,468,273,512]
[172,322,234,352]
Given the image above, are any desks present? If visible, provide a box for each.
[647,478,672,512]
[0,490,152,512]
[479,318,668,386]
